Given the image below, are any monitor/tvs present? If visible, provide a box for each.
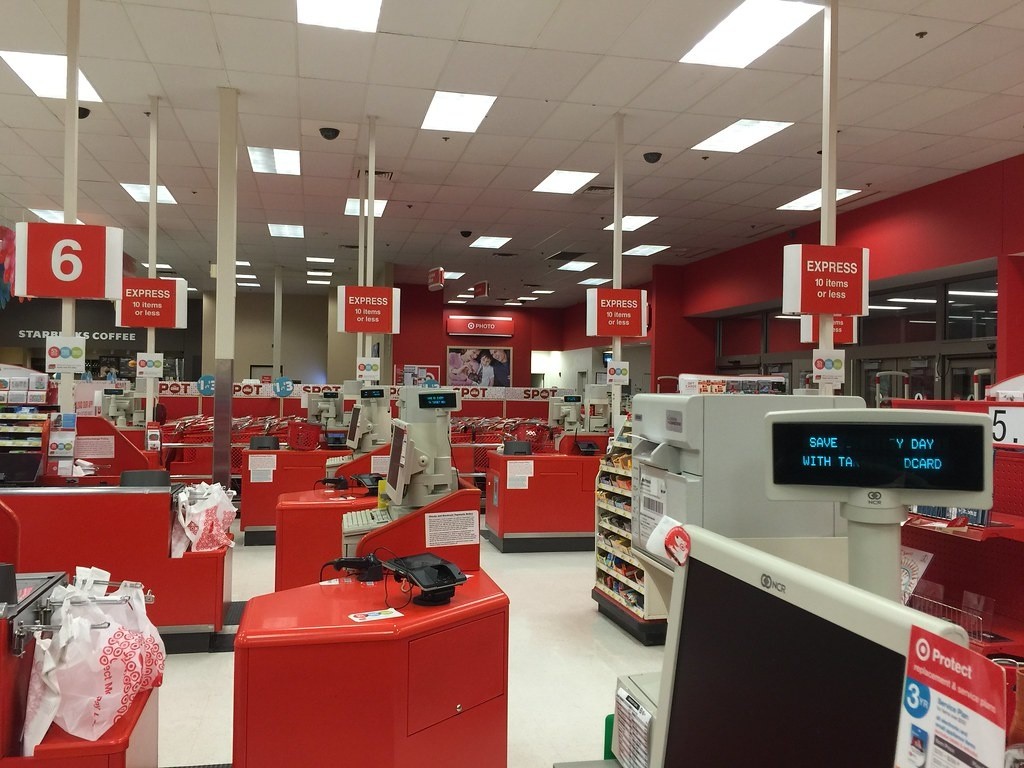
[386,418,414,504]
[307,394,344,425]
[350,473,384,488]
[645,523,969,768]
[548,397,582,427]
[101,390,135,429]
[385,552,467,591]
[346,404,364,449]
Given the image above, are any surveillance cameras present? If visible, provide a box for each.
[318,128,340,140]
[460,231,472,237]
[817,151,822,154]
[78,107,90,119]
[643,152,662,163]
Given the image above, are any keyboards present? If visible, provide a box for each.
[327,455,352,468]
[343,506,392,536]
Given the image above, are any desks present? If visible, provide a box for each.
[0,401,1024,768]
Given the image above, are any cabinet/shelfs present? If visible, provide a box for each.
[0,373,60,454]
[587,414,674,646]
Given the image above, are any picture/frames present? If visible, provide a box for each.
[394,365,440,387]
[445,345,514,387]
[249,365,284,384]
